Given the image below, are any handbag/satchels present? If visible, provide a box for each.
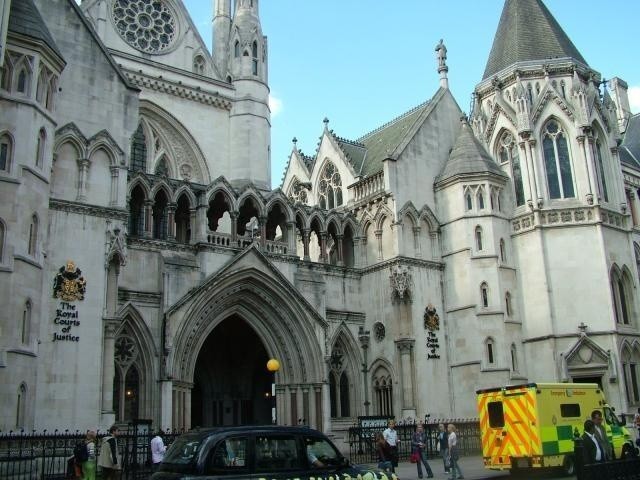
[446,456,451,467]
[436,439,440,452]
[410,452,421,463]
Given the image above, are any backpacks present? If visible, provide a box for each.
[73,440,91,463]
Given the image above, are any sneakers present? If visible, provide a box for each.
[418,472,464,479]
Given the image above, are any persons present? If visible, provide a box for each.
[149,430,168,469]
[579,420,605,463]
[435,38,448,66]
[591,410,611,461]
[632,407,640,446]
[376,420,466,480]
[306,441,324,468]
[79,430,97,480]
[96,426,123,479]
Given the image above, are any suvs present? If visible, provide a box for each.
[150,424,402,480]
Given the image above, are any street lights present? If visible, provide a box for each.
[266,359,280,424]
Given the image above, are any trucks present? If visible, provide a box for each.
[475,381,634,479]
